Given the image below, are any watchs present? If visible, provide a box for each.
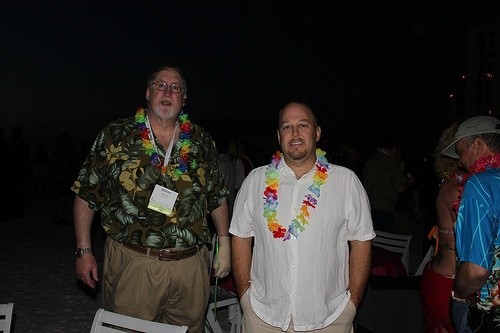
[74,247,90,257]
[451,290,469,304]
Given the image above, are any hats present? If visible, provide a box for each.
[457,117,500,141]
[437,125,465,158]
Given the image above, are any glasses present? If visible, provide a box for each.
[149,80,186,95]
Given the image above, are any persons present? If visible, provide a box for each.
[0,127,441,276]
[227,101,376,333]
[71,64,231,333]
[420,115,500,333]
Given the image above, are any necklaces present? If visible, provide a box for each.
[451,176,466,222]
[264,148,328,241]
[134,107,191,177]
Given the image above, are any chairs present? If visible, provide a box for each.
[371,229,412,277]
[90,307,188,333]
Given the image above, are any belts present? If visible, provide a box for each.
[107,235,205,261]
